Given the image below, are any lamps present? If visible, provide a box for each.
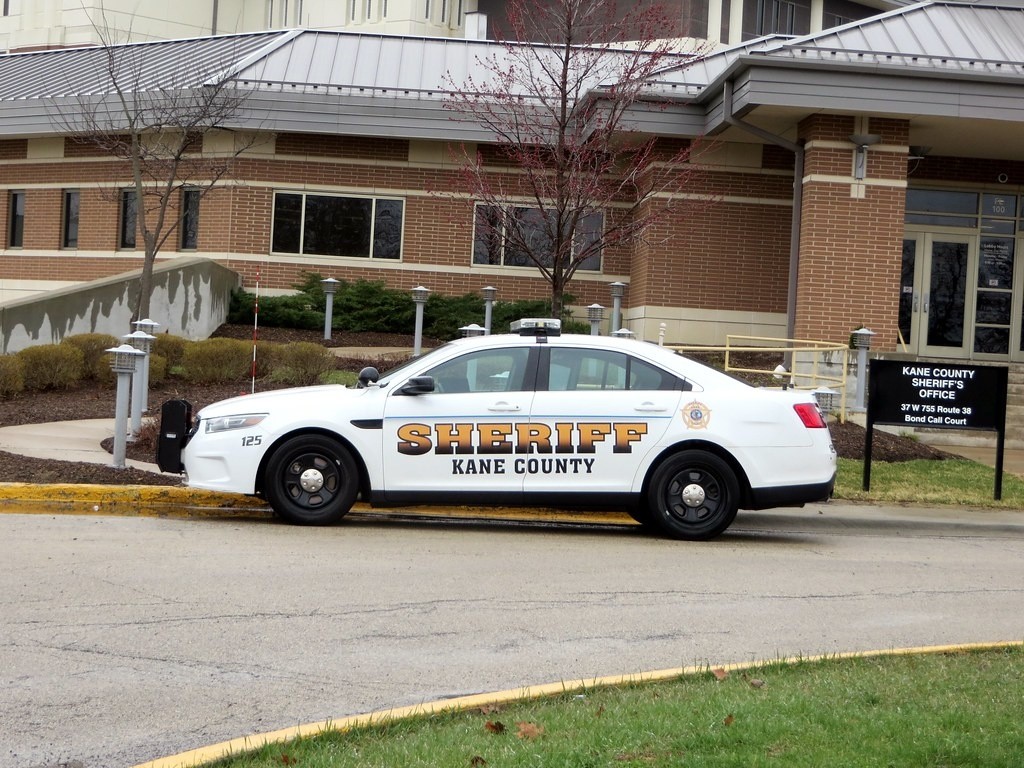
[849,134,881,167]
[584,304,606,321]
[459,324,490,337]
[907,145,932,175]
[105,345,146,373]
[480,286,499,300]
[609,282,628,297]
[321,278,341,293]
[610,328,638,340]
[123,318,161,356]
[850,328,877,347]
[410,286,433,302]
[815,387,841,412]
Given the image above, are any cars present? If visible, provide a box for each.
[157,318,837,541]
